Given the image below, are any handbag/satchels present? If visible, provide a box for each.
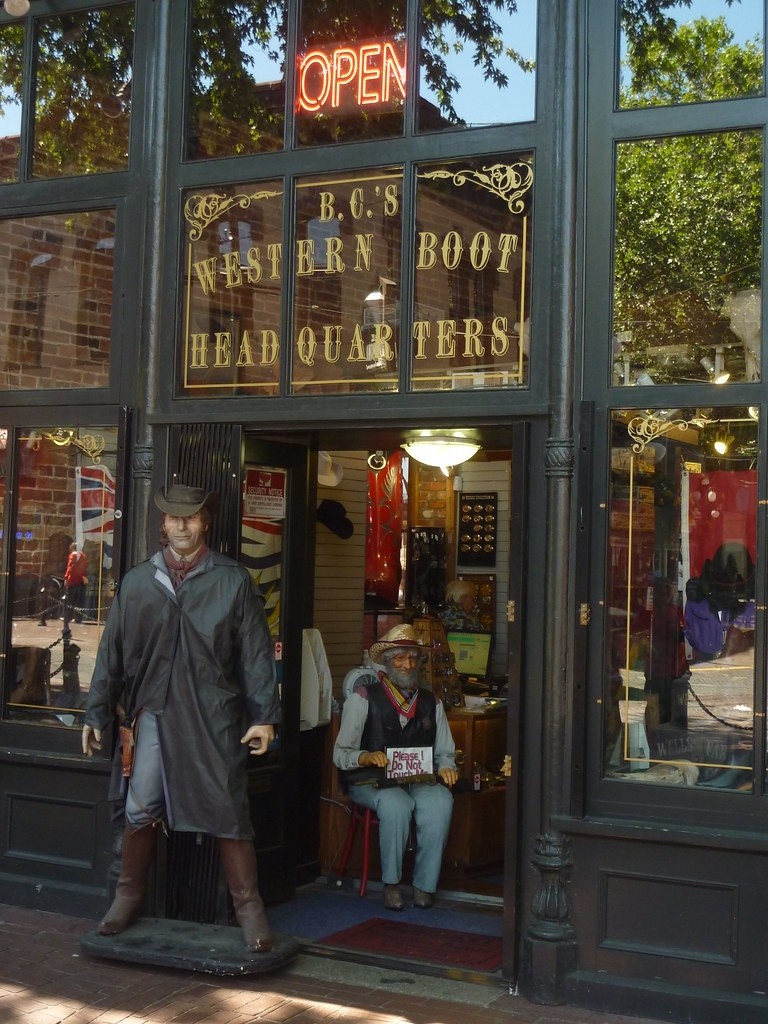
[82,576,89,585]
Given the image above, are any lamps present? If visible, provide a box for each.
[366,449,387,475]
[714,432,735,455]
[365,274,388,301]
[399,423,484,470]
[699,350,732,385]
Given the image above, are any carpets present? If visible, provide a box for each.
[314,918,502,969]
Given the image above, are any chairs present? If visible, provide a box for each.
[332,704,416,897]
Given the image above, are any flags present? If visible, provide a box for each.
[76,465,117,569]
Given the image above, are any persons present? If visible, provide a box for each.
[79,485,283,952]
[60,542,89,622]
[333,622,459,910]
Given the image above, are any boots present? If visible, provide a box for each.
[219,833,272,951]
[99,818,155,933]
[695,731,755,788]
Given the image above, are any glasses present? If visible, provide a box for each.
[466,594,477,603]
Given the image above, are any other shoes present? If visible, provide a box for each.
[412,884,433,909]
[71,619,82,623]
[382,882,404,909]
[60,616,65,620]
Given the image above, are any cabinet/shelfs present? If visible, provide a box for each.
[328,696,508,877]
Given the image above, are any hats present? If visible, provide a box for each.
[368,623,433,665]
[153,484,221,517]
